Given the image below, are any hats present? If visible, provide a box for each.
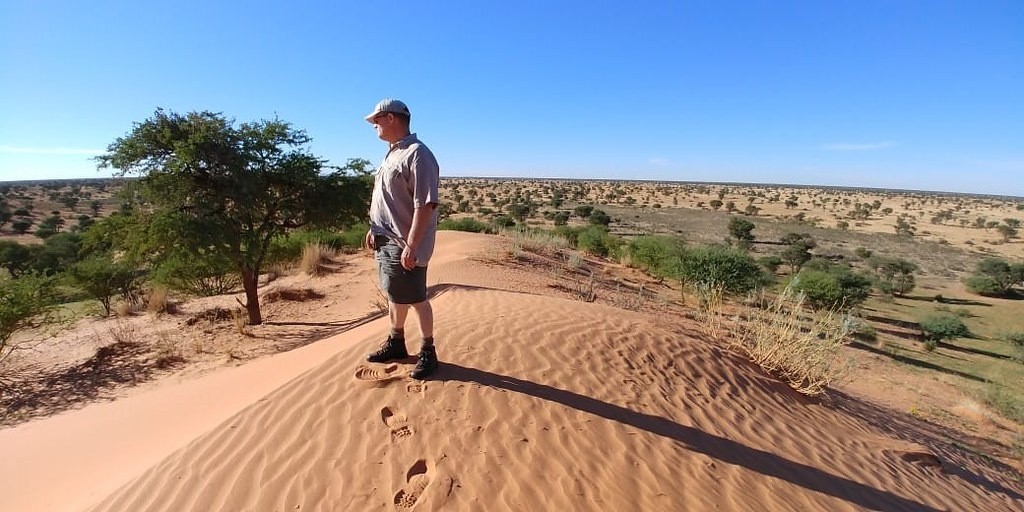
[365,100,411,123]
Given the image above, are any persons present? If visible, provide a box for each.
[363,98,439,381]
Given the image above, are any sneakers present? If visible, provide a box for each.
[367,337,410,361]
[410,347,438,378]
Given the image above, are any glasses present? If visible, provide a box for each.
[373,113,398,123]
